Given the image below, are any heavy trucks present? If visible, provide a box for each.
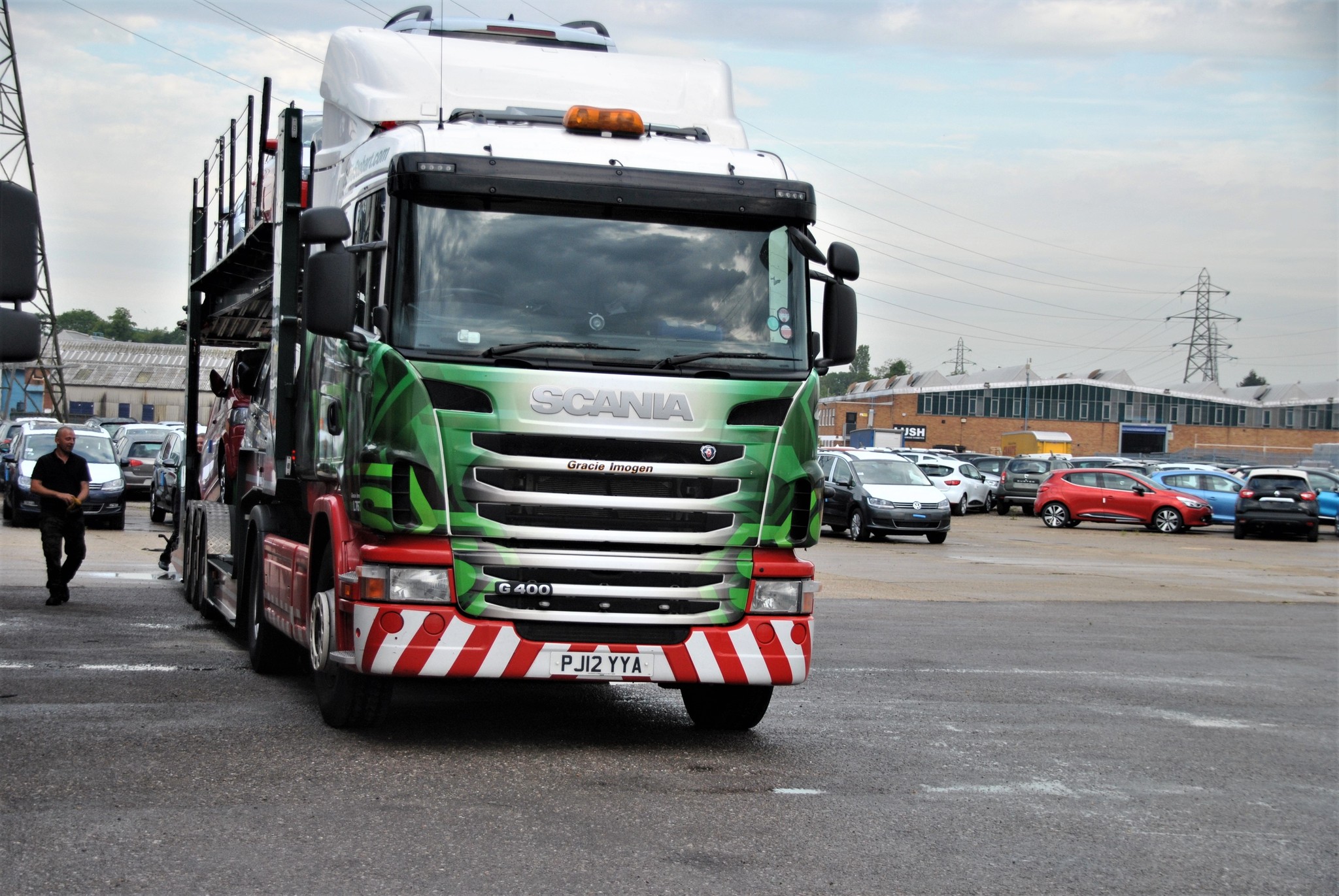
[174,2,860,728]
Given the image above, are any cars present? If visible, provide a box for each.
[0,416,210,445]
[111,433,174,501]
[1128,469,1247,531]
[1285,468,1339,538]
[237,325,303,448]
[818,447,1339,498]
[150,428,207,524]
[1033,468,1214,534]
[1,421,131,531]
[995,457,1076,516]
[1232,468,1321,543]
[817,447,951,545]
[907,459,993,516]
[214,5,620,263]
[189,353,255,507]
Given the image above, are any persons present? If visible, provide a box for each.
[158,434,204,571]
[30,426,92,606]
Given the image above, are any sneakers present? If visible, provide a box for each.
[46,589,61,605]
[59,583,70,601]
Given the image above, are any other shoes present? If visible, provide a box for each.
[158,561,169,571]
